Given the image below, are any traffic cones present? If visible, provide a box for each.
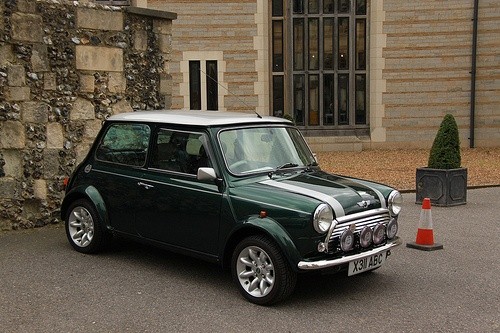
[407,196,444,256]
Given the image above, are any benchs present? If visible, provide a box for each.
[103,151,146,167]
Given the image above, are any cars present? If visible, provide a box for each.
[61,105,404,309]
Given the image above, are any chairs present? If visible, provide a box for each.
[156,143,187,173]
[198,141,227,167]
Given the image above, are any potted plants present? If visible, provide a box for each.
[415,114,467,207]
